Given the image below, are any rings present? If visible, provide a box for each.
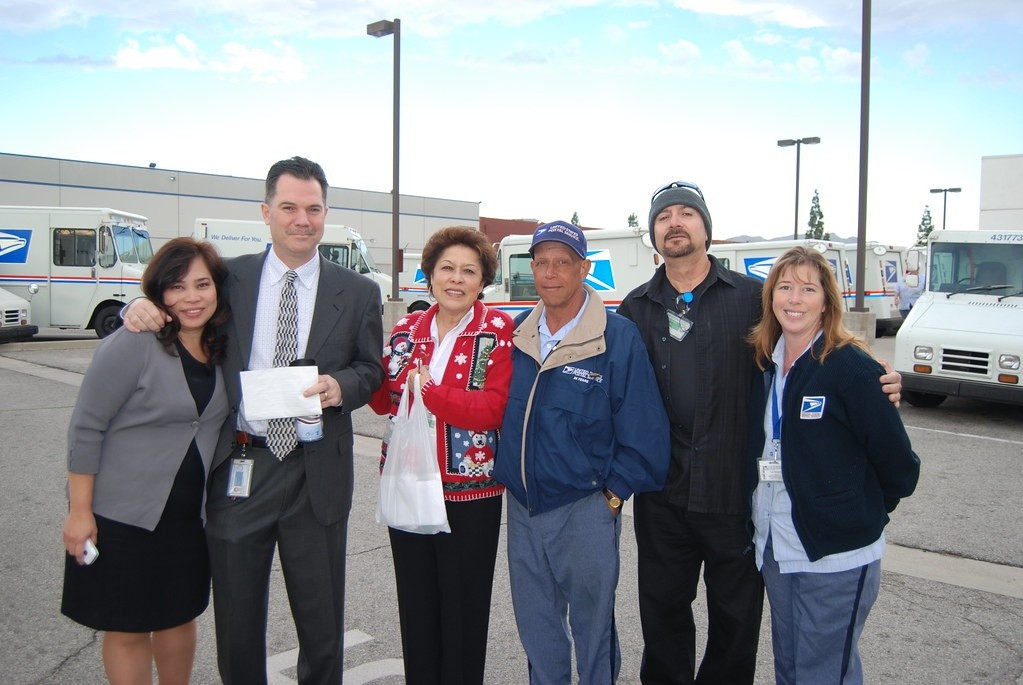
[324,392,328,399]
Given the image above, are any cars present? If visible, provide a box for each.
[0,282,42,343]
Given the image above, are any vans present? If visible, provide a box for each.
[193,217,393,314]
[893,228,1023,409]
[491,229,665,329]
[398,253,497,313]
[0,205,154,340]
[706,238,970,338]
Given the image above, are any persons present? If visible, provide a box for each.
[366,225,513,685]
[120,155,385,685]
[616,182,902,685]
[492,220,670,685]
[60,238,230,685]
[741,246,920,685]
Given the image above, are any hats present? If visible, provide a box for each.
[648,183,712,255]
[529,220,587,260]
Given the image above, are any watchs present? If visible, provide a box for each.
[601,487,623,513]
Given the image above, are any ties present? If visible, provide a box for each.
[265,270,300,461]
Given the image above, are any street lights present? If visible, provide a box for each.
[776,136,822,240]
[365,18,404,303]
[930,187,962,230]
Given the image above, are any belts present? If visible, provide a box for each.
[234,430,304,451]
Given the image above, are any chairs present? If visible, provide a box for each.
[972,261,1007,295]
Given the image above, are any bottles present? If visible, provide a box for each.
[289,358,324,443]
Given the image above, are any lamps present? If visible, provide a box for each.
[150,163,157,169]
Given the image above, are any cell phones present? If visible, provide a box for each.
[82,537,99,565]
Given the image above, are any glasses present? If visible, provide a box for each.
[650,181,705,201]
[671,293,693,333]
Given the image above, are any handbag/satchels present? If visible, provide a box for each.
[374,373,452,536]
